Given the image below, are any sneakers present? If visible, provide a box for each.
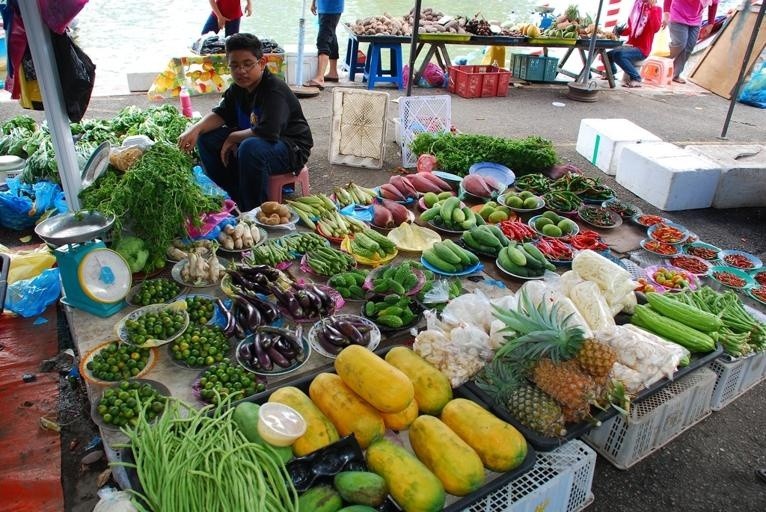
[673,77,685,83]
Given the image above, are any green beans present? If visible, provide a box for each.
[107,390,299,511]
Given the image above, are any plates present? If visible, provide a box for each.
[497,193,545,212]
[316,221,370,241]
[79,339,159,383]
[421,255,480,276]
[365,265,426,296]
[540,195,584,214]
[744,267,766,296]
[241,251,293,270]
[535,242,580,266]
[528,215,580,239]
[495,257,544,280]
[428,220,465,233]
[718,249,764,269]
[236,327,311,376]
[496,223,540,244]
[683,242,722,262]
[373,186,413,204]
[644,264,698,291]
[419,196,465,211]
[171,258,226,287]
[640,238,682,256]
[708,266,753,289]
[748,285,766,305]
[277,283,344,323]
[192,366,267,405]
[124,281,188,307]
[218,226,268,253]
[431,171,463,184]
[602,200,643,218]
[469,162,516,187]
[80,142,111,190]
[280,232,331,256]
[665,254,713,276]
[362,295,423,331]
[308,314,381,360]
[300,248,357,278]
[470,204,517,225]
[586,185,616,203]
[366,209,415,231]
[633,214,674,228]
[387,228,441,251]
[249,206,299,231]
[341,237,398,264]
[648,222,690,244]
[579,203,623,229]
[220,293,283,338]
[90,378,174,430]
[221,264,288,299]
[176,293,230,332]
[342,204,374,222]
[165,342,238,370]
[166,237,219,263]
[327,273,374,302]
[117,303,189,347]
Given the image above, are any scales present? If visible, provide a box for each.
[534,6,556,31]
[34,208,133,318]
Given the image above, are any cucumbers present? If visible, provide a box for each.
[349,229,396,258]
[460,225,507,254]
[419,238,479,271]
[496,240,556,276]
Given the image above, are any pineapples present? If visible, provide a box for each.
[476,290,617,436]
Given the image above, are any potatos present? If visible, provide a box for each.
[351,7,467,36]
[256,201,292,224]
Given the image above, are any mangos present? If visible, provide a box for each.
[540,24,578,38]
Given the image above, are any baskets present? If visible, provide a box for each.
[629,242,726,291]
[400,95,452,170]
[580,367,717,469]
[462,438,597,512]
[709,305,766,412]
[392,118,462,148]
[327,87,388,169]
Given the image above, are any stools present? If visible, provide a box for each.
[640,55,674,87]
[264,165,311,205]
[361,41,403,90]
[342,34,381,81]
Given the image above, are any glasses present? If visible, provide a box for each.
[228,58,261,72]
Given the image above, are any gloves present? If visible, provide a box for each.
[700,25,713,38]
[661,13,670,29]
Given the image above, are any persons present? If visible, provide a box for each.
[177,32,314,214]
[659,0,722,86]
[201,0,253,36]
[303,0,345,89]
[602,0,663,88]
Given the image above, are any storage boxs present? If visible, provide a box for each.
[573,116,660,176]
[614,140,720,210]
[683,141,766,208]
[282,49,318,84]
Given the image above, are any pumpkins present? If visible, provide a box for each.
[380,402,419,431]
[336,346,415,411]
[370,445,447,512]
[411,413,485,494]
[441,397,528,470]
[308,373,385,446]
[230,386,339,465]
[384,348,454,413]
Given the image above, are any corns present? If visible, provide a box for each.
[420,197,478,230]
[318,212,366,238]
[286,194,336,218]
[333,182,377,205]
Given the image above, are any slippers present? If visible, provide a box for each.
[324,75,339,82]
[601,76,617,80]
[303,80,325,91]
[622,81,643,87]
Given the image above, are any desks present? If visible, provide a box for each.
[410,31,626,91]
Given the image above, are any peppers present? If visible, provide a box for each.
[503,221,536,243]
[536,238,572,261]
[515,173,549,195]
[545,190,582,212]
[584,185,611,201]
[569,231,607,251]
[550,175,590,193]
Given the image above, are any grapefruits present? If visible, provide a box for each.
[520,23,538,37]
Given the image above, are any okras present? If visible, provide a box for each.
[304,247,357,274]
[277,233,326,253]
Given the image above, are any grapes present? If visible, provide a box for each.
[466,19,490,35]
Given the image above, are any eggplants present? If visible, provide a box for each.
[217,293,280,338]
[238,332,302,370]
[335,469,385,507]
[338,505,378,512]
[297,485,340,512]
[279,281,333,318]
[224,264,278,294]
[317,320,370,354]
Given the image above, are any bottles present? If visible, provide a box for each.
[179,86,193,119]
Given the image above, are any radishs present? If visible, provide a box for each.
[177,253,224,283]
[219,224,261,249]
[167,239,217,259]
